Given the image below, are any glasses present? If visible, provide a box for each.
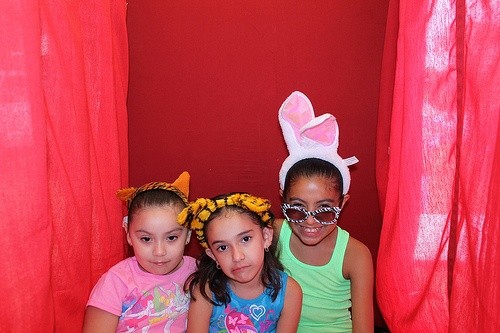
[281,203,341,225]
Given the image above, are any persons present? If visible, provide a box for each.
[178,193,303,333]
[273,91,374,333]
[82,171,200,333]
